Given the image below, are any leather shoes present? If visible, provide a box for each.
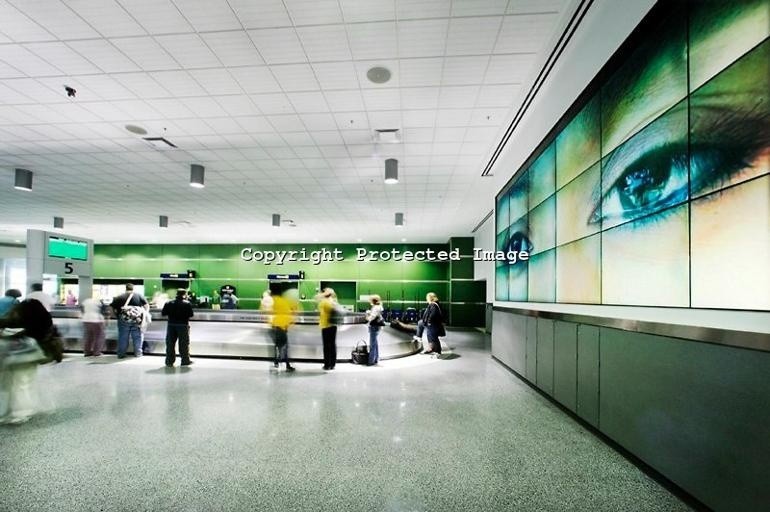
[323,365,335,370]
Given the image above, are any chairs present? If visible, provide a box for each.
[382,306,426,323]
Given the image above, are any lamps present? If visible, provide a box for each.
[13,169,33,192]
[189,163,205,188]
[53,217,64,230]
[384,159,399,184]
[159,215,168,228]
[394,213,405,227]
[271,213,280,228]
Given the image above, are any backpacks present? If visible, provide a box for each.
[328,308,344,326]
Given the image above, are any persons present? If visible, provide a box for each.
[81,298,108,357]
[260,283,300,372]
[110,284,147,357]
[66,289,77,304]
[314,289,341,369]
[25,282,50,314]
[495,0,768,316]
[415,292,444,355]
[161,289,194,367]
[364,295,385,365]
[0,289,20,317]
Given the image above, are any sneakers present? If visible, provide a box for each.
[181,361,193,366]
[167,363,173,367]
[118,355,127,359]
[366,361,378,366]
[84,351,104,357]
[273,361,295,372]
[430,352,441,360]
[413,335,422,342]
[135,348,143,356]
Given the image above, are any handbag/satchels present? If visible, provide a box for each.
[355,340,367,352]
[370,316,385,326]
[352,351,370,364]
[438,324,446,337]
[119,306,144,326]
